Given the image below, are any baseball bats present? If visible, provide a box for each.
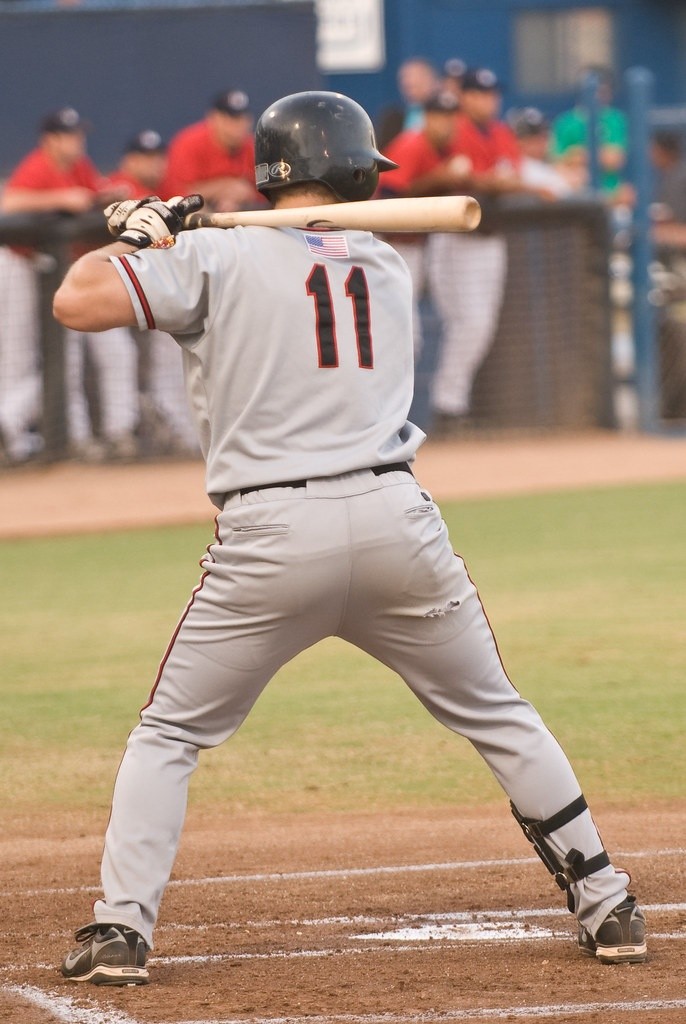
[182,194,482,234]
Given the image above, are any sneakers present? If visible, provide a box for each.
[60,922,149,987]
[578,896,647,963]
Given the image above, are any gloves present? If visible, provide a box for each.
[115,194,204,248]
[103,195,162,237]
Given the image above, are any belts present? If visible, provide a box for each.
[241,462,414,499]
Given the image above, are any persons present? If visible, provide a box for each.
[0,58,686,469]
[52,90,647,988]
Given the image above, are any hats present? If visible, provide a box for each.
[41,108,87,132]
[122,130,168,156]
[212,91,249,116]
[464,70,499,91]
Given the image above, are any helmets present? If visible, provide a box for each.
[254,91,399,201]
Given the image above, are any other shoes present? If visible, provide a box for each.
[110,434,140,465]
[72,437,108,464]
